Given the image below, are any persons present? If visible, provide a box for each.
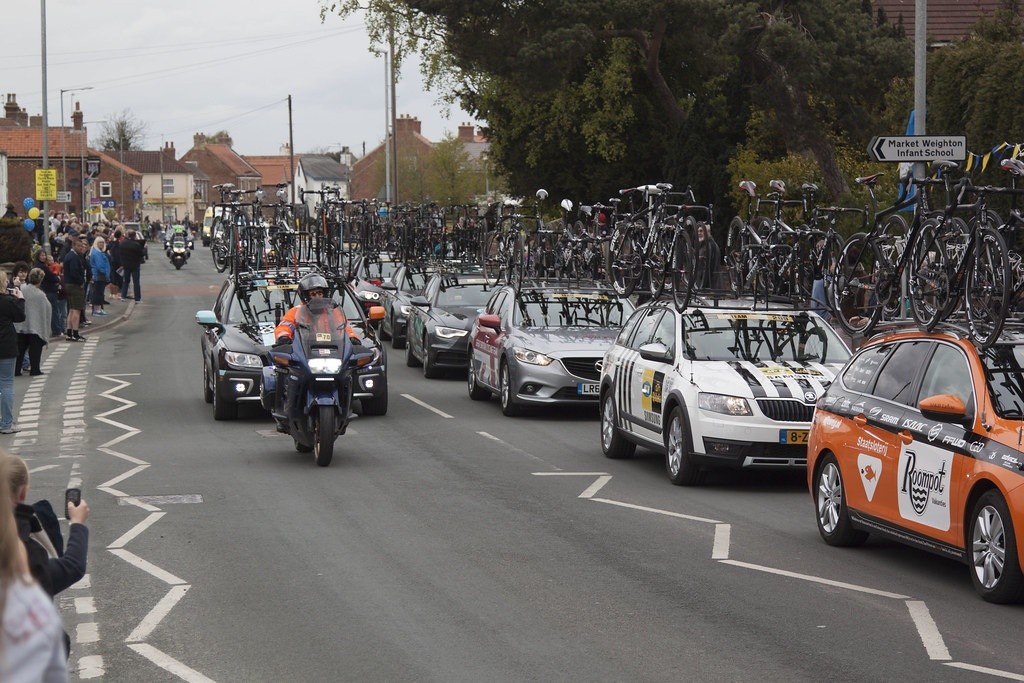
[166,225,190,259]
[33,209,149,307]
[7,261,29,295]
[13,267,52,376]
[88,236,110,316]
[0,446,91,683]
[811,256,854,323]
[32,250,64,337]
[274,272,361,419]
[697,222,720,288]
[149,219,203,241]
[45,255,61,293]
[119,231,145,304]
[62,239,88,342]
[0,270,26,434]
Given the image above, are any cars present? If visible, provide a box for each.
[163,225,194,250]
[37,217,61,232]
[233,220,297,266]
[406,273,508,378]
[322,250,360,278]
[381,263,439,348]
[347,251,406,327]
[467,275,641,417]
[196,262,387,420]
[808,311,1023,604]
[598,290,857,487]
[133,231,149,259]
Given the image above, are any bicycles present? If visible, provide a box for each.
[209,140,1024,349]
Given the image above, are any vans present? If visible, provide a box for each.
[203,206,234,247]
[123,222,142,233]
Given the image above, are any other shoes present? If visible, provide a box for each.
[135,300,144,304]
[65,335,72,341]
[112,294,122,299]
[95,311,108,317]
[109,295,112,298]
[15,372,22,377]
[277,423,284,432]
[31,371,44,375]
[92,312,96,317]
[72,335,86,342]
[83,320,92,324]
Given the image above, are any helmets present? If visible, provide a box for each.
[176,229,183,234]
[298,273,330,304]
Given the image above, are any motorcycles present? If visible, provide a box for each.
[258,299,384,466]
[169,234,189,270]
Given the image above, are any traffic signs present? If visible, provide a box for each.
[867,133,965,163]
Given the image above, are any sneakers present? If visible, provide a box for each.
[0,421,21,433]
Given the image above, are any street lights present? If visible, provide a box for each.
[80,121,109,223]
[61,88,94,211]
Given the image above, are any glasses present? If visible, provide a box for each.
[311,291,323,296]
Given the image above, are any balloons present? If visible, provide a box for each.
[24,219,35,231]
[29,207,39,219]
[23,197,34,210]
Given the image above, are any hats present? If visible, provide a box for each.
[68,228,80,237]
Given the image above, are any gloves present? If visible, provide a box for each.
[350,337,361,346]
[278,336,293,346]
